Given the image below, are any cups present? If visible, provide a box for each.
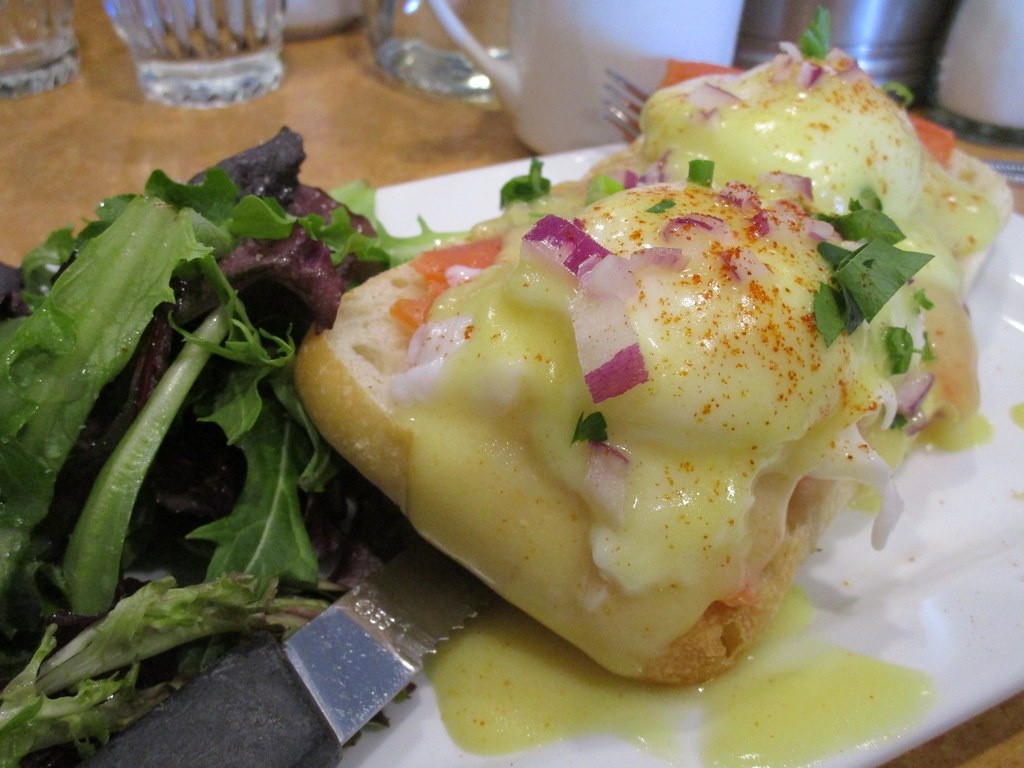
[101,0,287,110]
[426,1,743,156]
[1,0,83,98]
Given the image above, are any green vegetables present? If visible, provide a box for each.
[0,4,941,768]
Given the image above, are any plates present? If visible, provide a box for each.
[330,142,1023,768]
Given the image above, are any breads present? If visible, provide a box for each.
[290,134,877,690]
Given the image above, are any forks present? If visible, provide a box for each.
[602,66,1023,184]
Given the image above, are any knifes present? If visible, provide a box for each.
[75,539,496,767]
